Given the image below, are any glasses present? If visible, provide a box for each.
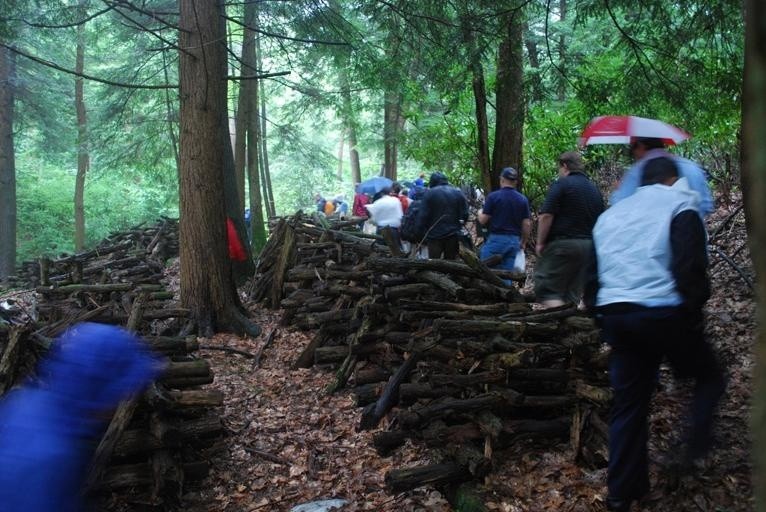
[629,138,642,149]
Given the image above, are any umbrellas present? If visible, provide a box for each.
[576,113,689,148]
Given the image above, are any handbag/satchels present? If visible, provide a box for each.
[400,208,419,243]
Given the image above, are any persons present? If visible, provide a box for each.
[0,320,167,512]
[476,168,531,291]
[535,152,605,319]
[609,136,714,226]
[352,172,468,263]
[584,156,726,510]
[315,191,348,217]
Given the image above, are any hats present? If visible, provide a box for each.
[502,167,517,180]
[430,172,448,187]
